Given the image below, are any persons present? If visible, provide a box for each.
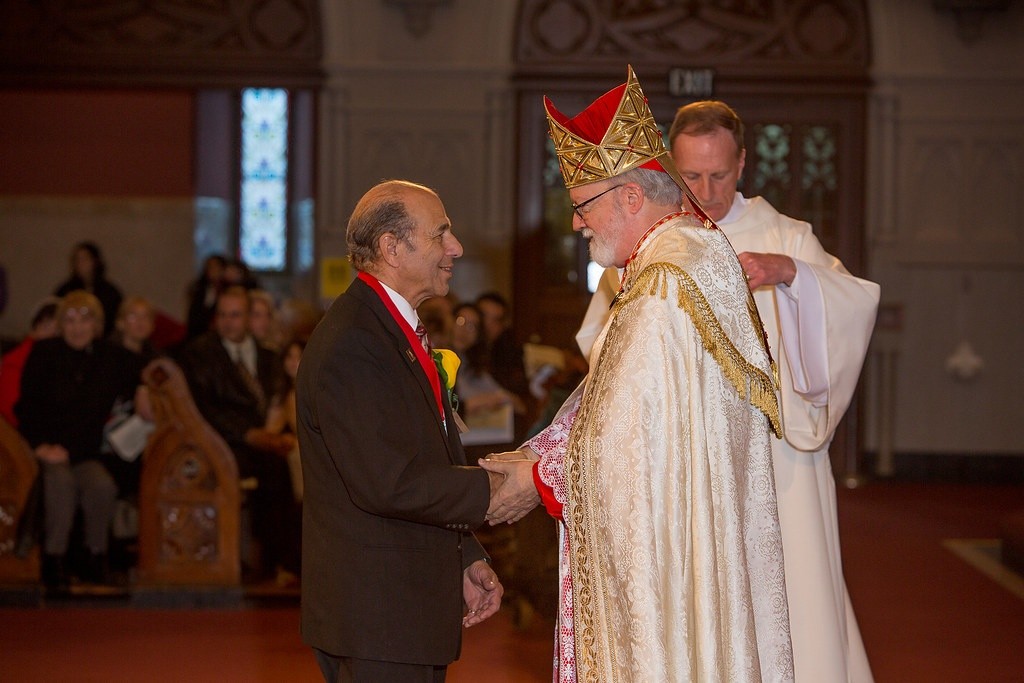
[477,63,795,683]
[574,100,881,683]
[416,290,538,457]
[293,181,509,683]
[0,240,326,611]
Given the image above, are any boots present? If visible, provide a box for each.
[42,551,73,601]
[85,546,129,586]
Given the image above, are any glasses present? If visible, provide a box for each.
[571,185,622,219]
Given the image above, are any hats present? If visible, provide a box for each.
[543,65,669,189]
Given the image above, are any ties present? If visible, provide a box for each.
[232,347,265,413]
[415,320,432,361]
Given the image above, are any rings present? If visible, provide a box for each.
[746,274,750,281]
[470,612,475,614]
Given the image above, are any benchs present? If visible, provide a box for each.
[2,310,261,599]
